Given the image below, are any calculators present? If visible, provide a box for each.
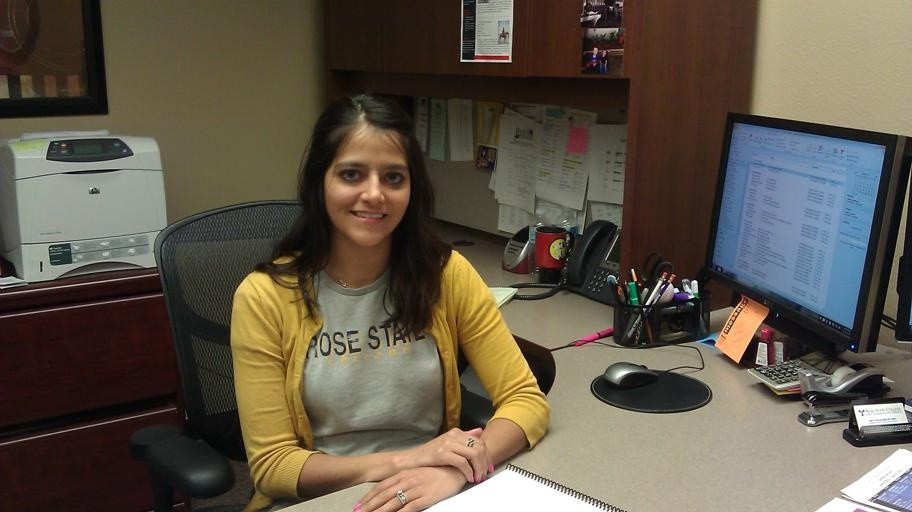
[748,351,846,391]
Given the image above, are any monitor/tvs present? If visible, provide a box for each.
[702,109,912,353]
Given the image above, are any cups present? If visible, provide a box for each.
[534,225,574,286]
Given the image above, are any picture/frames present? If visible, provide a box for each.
[0,0,108,118]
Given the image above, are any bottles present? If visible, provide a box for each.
[754,326,777,370]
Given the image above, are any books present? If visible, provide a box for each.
[422,462,627,512]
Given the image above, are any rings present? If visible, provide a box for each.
[397,490,407,506]
[464,435,476,447]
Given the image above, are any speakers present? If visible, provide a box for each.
[501,224,533,274]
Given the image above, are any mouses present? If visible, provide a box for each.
[604,359,657,388]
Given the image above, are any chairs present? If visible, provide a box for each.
[128,200,498,512]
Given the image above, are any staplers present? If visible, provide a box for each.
[798,391,869,427]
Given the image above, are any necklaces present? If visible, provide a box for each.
[336,272,355,288]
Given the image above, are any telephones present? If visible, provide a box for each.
[564,220,622,306]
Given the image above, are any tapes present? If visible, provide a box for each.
[831,366,857,387]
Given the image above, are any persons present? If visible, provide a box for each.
[598,50,607,72]
[589,48,598,73]
[230,94,551,511]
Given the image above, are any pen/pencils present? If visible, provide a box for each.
[607,269,709,346]
[575,328,614,346]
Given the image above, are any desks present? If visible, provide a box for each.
[436,219,912,512]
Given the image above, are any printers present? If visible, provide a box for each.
[0,130,167,281]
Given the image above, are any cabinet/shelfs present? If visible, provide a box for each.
[0,257,192,512]
[322,0,762,312]
